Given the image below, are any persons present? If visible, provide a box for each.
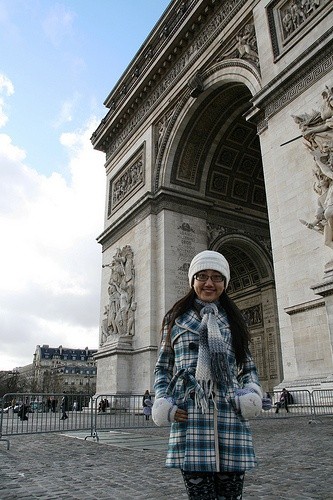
[153,250,263,500]
[302,88,333,250]
[19,391,109,421]
[142,390,151,420]
[99,249,136,347]
[275,388,294,414]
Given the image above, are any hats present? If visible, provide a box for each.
[188,250,231,292]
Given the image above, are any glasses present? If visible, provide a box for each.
[194,274,226,282]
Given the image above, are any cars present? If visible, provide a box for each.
[3,405,22,413]
